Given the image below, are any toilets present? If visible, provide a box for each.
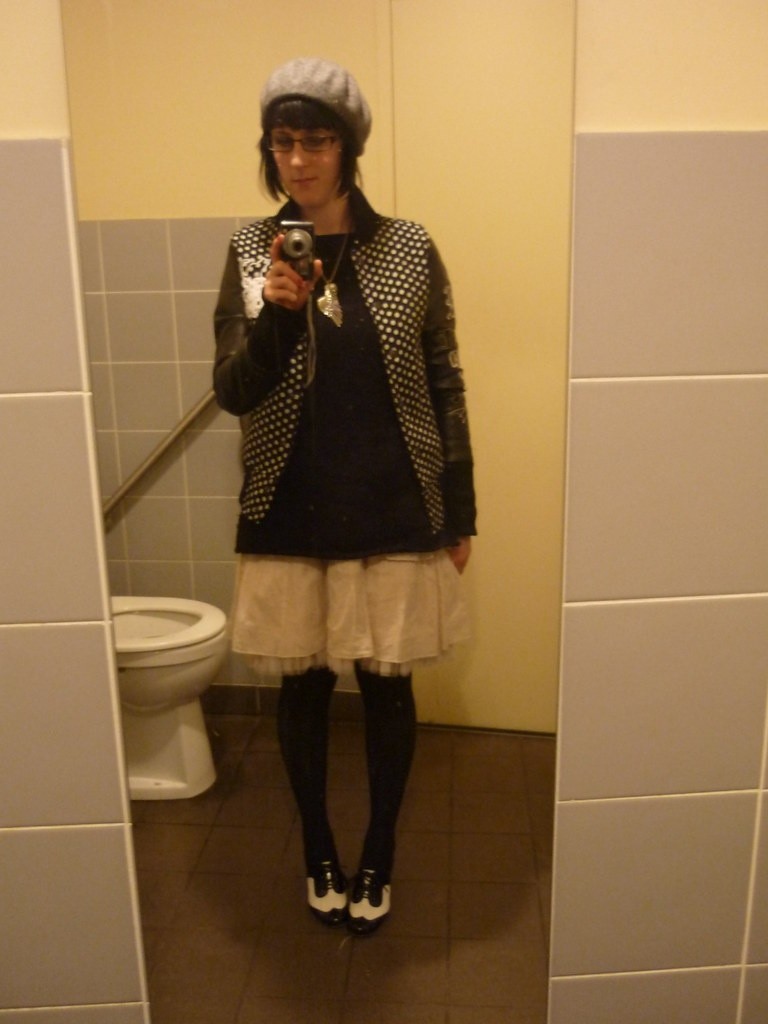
[108,595,228,799]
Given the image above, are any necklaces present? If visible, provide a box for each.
[317,229,349,327]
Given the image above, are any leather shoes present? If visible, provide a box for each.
[304,849,349,929]
[349,865,392,939]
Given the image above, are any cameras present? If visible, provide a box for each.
[280,220,315,282]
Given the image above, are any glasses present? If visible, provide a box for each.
[262,130,343,152]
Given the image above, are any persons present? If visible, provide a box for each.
[210,57,477,933]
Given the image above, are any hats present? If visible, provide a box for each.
[260,58,372,156]
